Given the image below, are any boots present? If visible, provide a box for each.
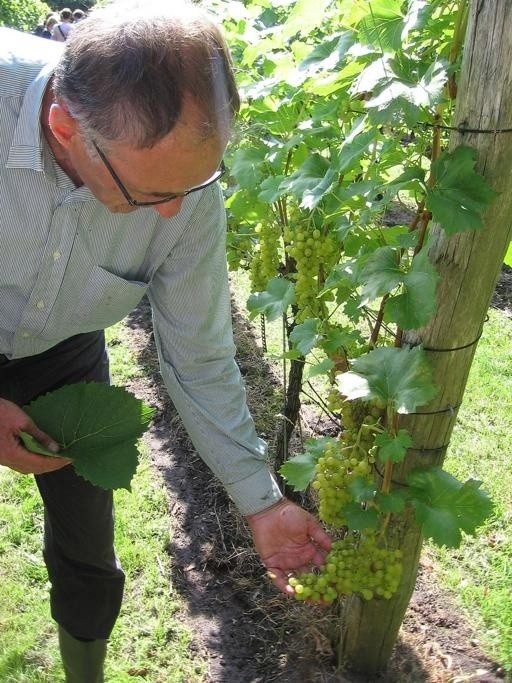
[58,624,107,682]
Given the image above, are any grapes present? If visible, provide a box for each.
[289,529,403,603]
[226,215,332,324]
[313,388,385,524]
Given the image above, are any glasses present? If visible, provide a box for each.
[90,141,228,209]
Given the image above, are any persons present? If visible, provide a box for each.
[1,0,332,683]
[33,6,86,42]
